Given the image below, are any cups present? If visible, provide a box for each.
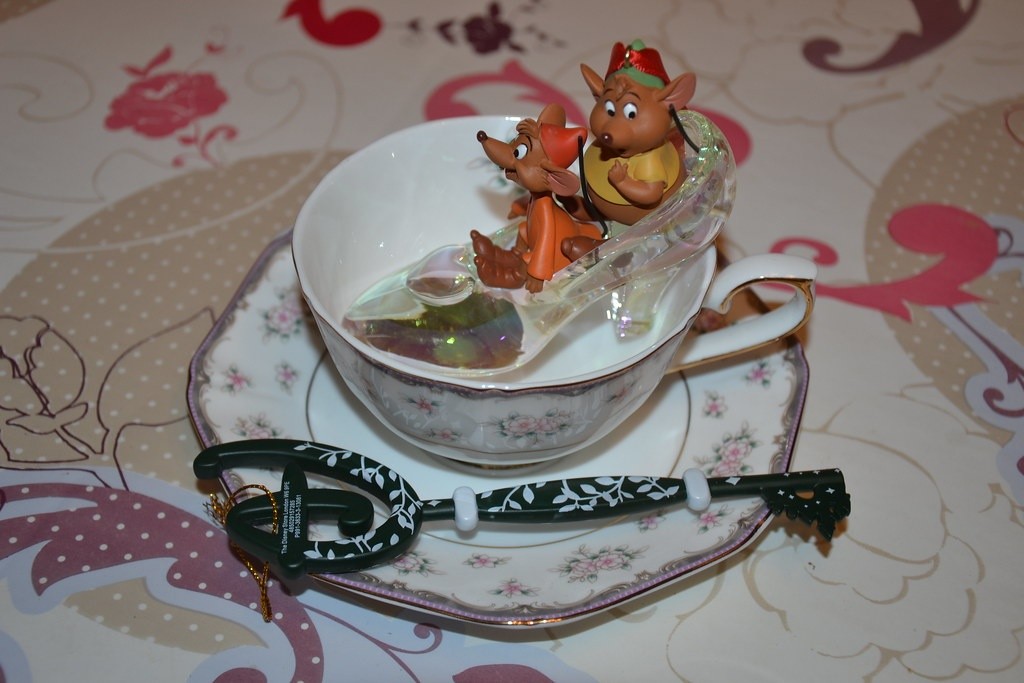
[290,113,815,468]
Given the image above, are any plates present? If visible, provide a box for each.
[186,230,810,631]
